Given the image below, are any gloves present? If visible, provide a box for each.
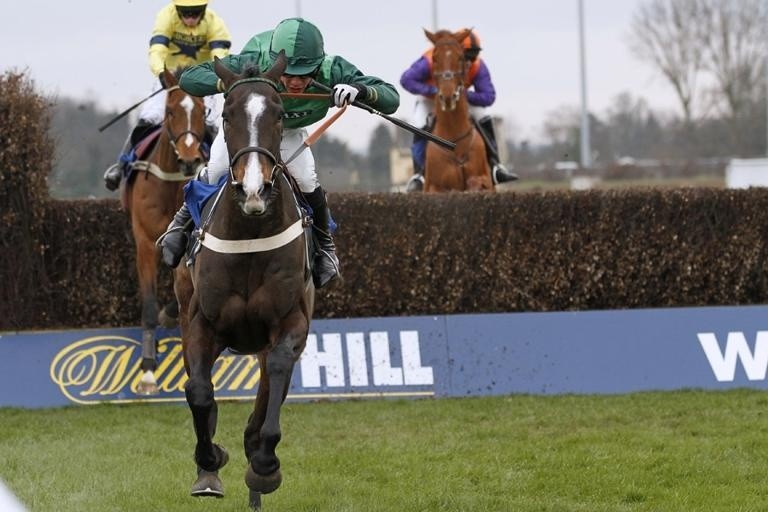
[329,83,367,108]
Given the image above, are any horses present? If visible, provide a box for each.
[421,23,492,194]
[125,59,212,396]
[155,43,319,499]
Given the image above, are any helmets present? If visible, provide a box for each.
[460,28,480,49]
[174,0,209,7]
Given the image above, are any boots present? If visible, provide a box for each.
[475,115,520,185]
[406,131,428,192]
[302,185,339,289]
[162,166,210,268]
[104,119,153,192]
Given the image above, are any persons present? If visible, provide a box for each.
[157,17,402,289]
[98,0,236,189]
[394,27,522,189]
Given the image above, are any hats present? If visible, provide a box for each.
[269,18,325,75]
